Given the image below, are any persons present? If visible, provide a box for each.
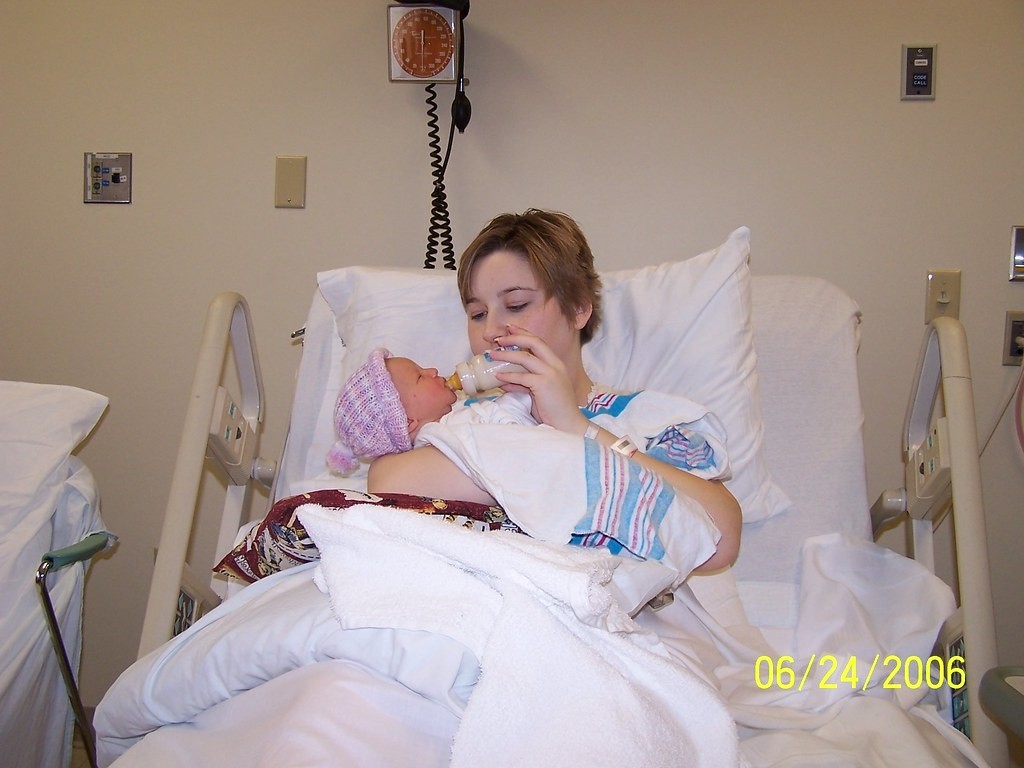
[326,344,536,447]
[366,205,741,571]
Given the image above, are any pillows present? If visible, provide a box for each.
[0,379,108,544]
[316,225,792,531]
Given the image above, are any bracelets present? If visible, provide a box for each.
[585,422,599,439]
[610,434,638,459]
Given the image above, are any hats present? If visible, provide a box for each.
[326,346,412,477]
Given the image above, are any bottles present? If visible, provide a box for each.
[443,345,523,395]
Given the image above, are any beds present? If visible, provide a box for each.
[88,266,1010,768]
[0,455,112,768]
